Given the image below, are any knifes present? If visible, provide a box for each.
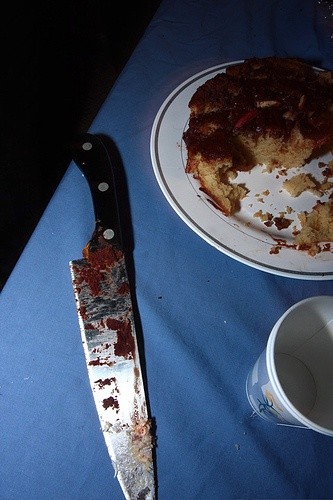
[64,131,158,500]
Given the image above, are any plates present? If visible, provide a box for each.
[150,57,332,281]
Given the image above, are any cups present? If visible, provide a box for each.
[243,296,333,435]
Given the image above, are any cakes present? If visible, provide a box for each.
[182,56,333,254]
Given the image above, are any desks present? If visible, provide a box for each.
[0,0,333,500]
